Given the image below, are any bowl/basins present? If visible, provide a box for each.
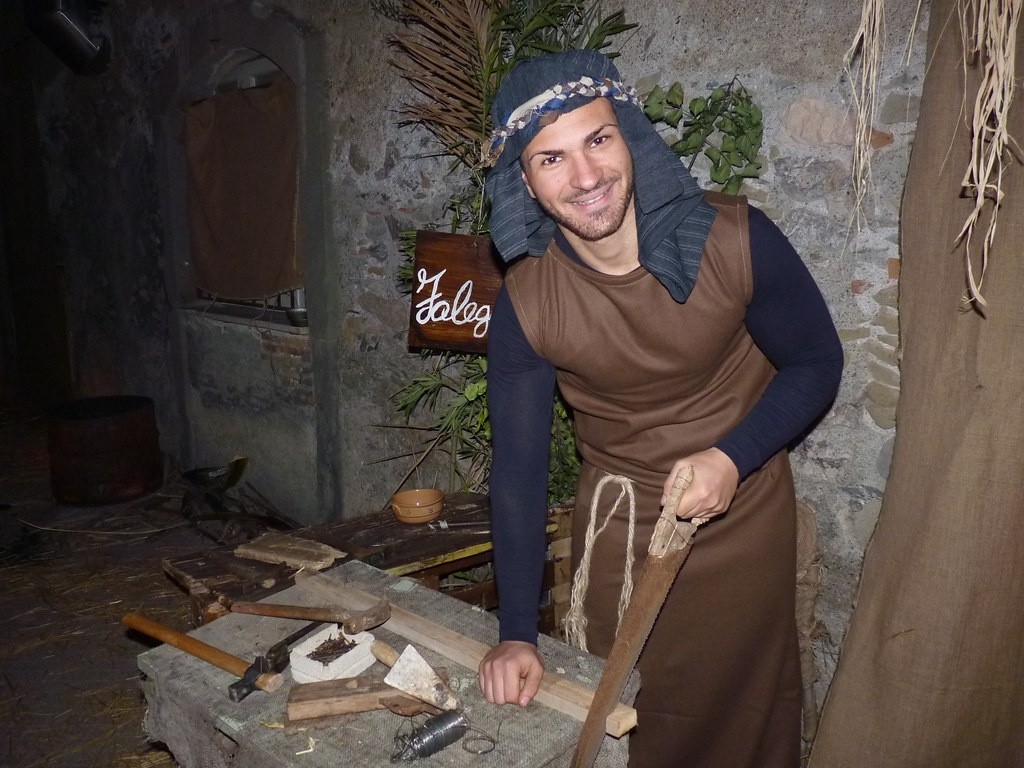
[391,488,444,524]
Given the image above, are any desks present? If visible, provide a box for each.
[161,491,560,635]
[137,558,642,768]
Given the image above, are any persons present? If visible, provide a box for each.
[476,49,844,768]
